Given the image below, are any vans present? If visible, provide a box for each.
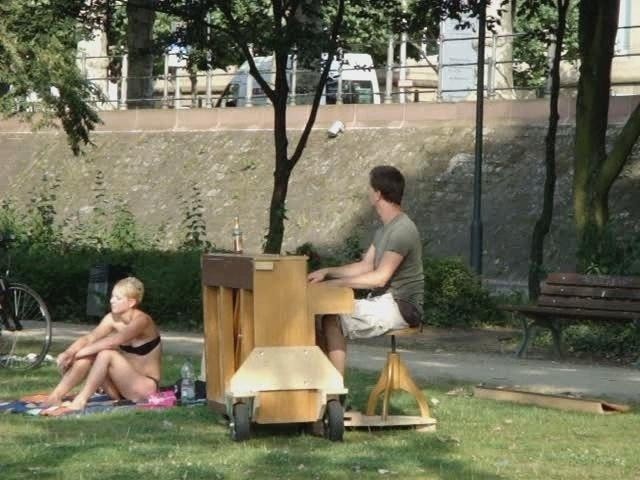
[214,53,381,107]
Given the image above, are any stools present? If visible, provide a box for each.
[367,324,430,422]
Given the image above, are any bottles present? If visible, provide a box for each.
[233,215,243,254]
[179,356,196,407]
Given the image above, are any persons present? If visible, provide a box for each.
[307,157,424,377]
[40,277,162,416]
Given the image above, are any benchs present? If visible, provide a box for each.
[496,272,640,360]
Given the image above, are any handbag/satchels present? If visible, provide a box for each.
[147,391,177,406]
[397,299,421,327]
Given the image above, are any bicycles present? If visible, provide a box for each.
[0,250,51,376]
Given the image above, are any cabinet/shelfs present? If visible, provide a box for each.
[199,251,354,414]
